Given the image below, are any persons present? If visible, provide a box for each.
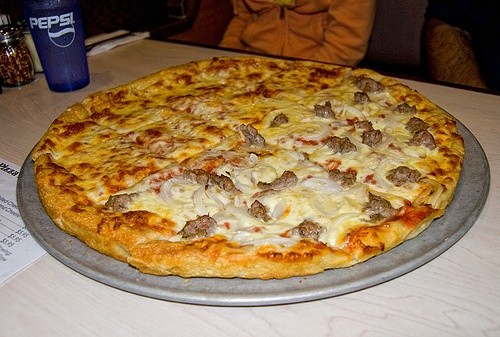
[218,1,376,67]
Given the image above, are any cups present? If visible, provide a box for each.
[19,0,96,93]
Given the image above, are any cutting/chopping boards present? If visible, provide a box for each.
[15,56,492,307]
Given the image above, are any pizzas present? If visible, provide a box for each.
[29,56,463,278]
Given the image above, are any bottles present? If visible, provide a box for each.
[0,24,35,90]
